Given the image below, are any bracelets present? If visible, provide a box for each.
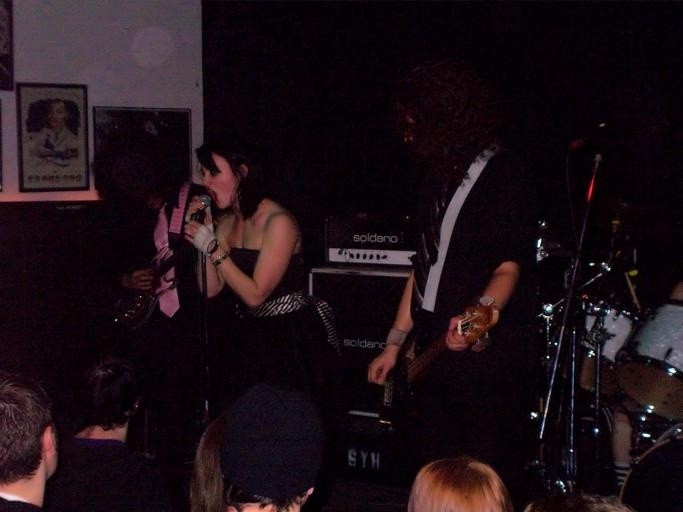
[205,237,230,266]
[385,326,406,346]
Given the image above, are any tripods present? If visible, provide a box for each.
[537,152,606,478]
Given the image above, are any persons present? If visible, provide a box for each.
[23,96,78,162]
[406,455,515,510]
[44,355,174,512]
[216,378,328,512]
[365,52,542,511]
[0,367,61,511]
[610,277,683,489]
[188,415,225,512]
[184,123,342,416]
[93,149,207,465]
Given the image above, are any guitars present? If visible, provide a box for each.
[381,296,496,419]
[113,211,231,330]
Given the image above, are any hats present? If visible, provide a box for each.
[220,382,324,499]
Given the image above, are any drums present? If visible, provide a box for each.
[557,304,638,394]
[613,300,683,423]
[618,426,682,511]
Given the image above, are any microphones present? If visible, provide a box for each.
[570,119,600,150]
[191,193,212,225]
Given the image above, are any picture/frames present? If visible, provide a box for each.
[13,81,89,193]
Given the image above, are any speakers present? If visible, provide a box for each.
[309,267,413,418]
[325,212,417,266]
